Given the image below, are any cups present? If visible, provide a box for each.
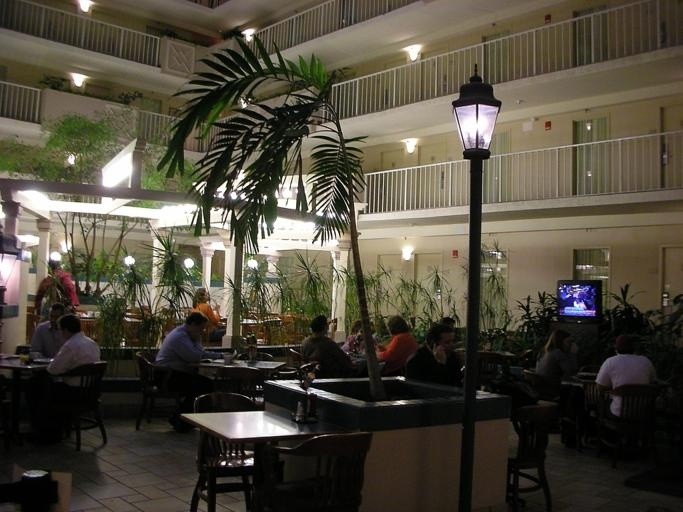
[223,353,232,365]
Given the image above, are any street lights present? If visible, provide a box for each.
[452,64,503,510]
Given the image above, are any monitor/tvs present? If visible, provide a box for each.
[555,278,602,321]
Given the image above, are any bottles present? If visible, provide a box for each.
[306,391,316,417]
[295,402,304,422]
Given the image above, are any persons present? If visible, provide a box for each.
[301,314,353,376]
[373,314,418,370]
[593,332,662,425]
[32,258,81,315]
[32,301,71,360]
[190,287,222,334]
[34,313,101,441]
[152,311,229,434]
[535,328,597,454]
[403,322,465,391]
[339,318,380,358]
[562,286,595,312]
